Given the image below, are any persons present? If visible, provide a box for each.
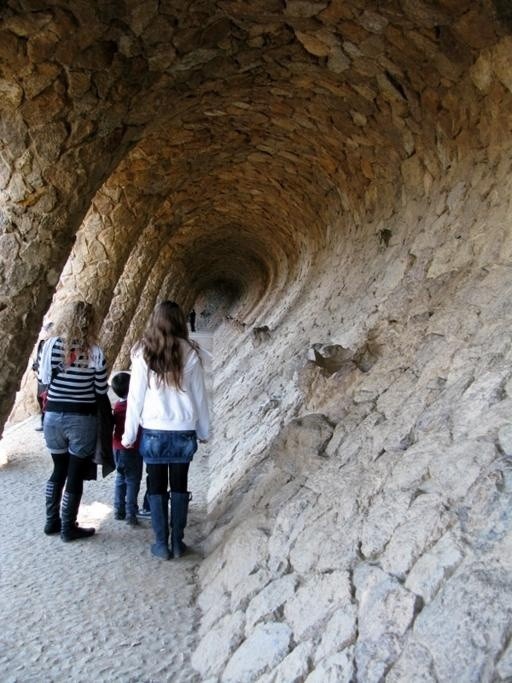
[111,300,212,560]
[189,308,196,332]
[30,322,56,431]
[32,300,113,542]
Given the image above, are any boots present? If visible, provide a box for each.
[44,481,95,542]
[150,493,189,559]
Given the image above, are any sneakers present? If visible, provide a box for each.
[113,508,151,527]
